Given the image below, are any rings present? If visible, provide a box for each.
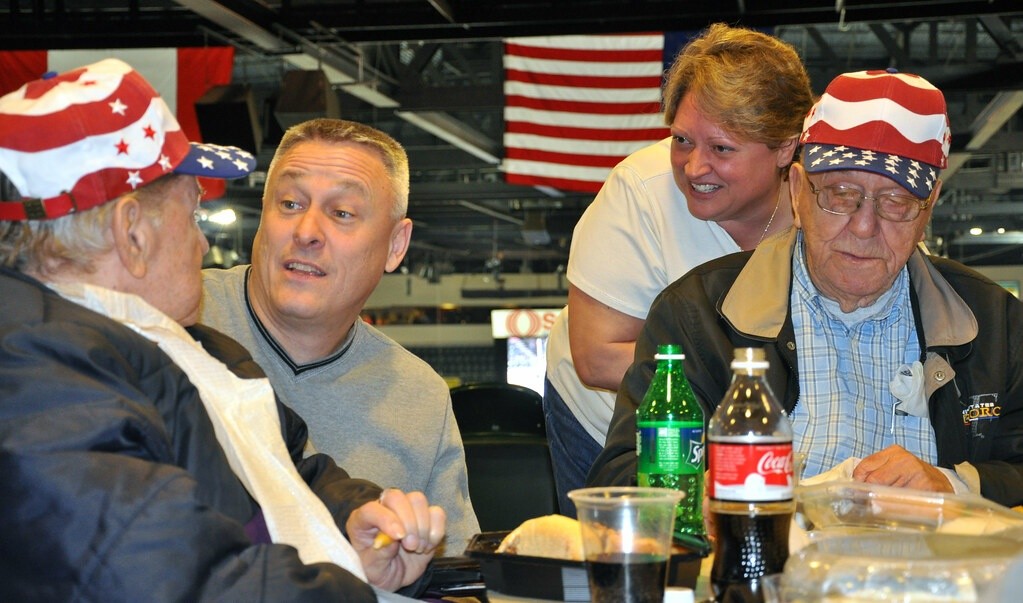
[379,487,398,504]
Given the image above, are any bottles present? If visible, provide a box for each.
[706,347,795,603]
[633,344,707,538]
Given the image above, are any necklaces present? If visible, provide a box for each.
[758,183,783,246]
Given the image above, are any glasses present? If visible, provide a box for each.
[807,173,936,221]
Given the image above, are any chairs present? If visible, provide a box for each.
[450,381,559,533]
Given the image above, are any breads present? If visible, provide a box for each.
[496,514,624,562]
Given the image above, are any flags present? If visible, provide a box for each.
[501,33,700,191]
[0,48,232,199]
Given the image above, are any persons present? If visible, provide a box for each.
[588,67,1023,525]
[543,28,816,524]
[0,56,447,603]
[194,119,482,559]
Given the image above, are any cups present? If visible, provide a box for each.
[566,486,685,602]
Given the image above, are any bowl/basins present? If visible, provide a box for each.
[466,526,712,600]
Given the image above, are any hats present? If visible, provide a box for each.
[797,68,952,199]
[0,59,258,220]
